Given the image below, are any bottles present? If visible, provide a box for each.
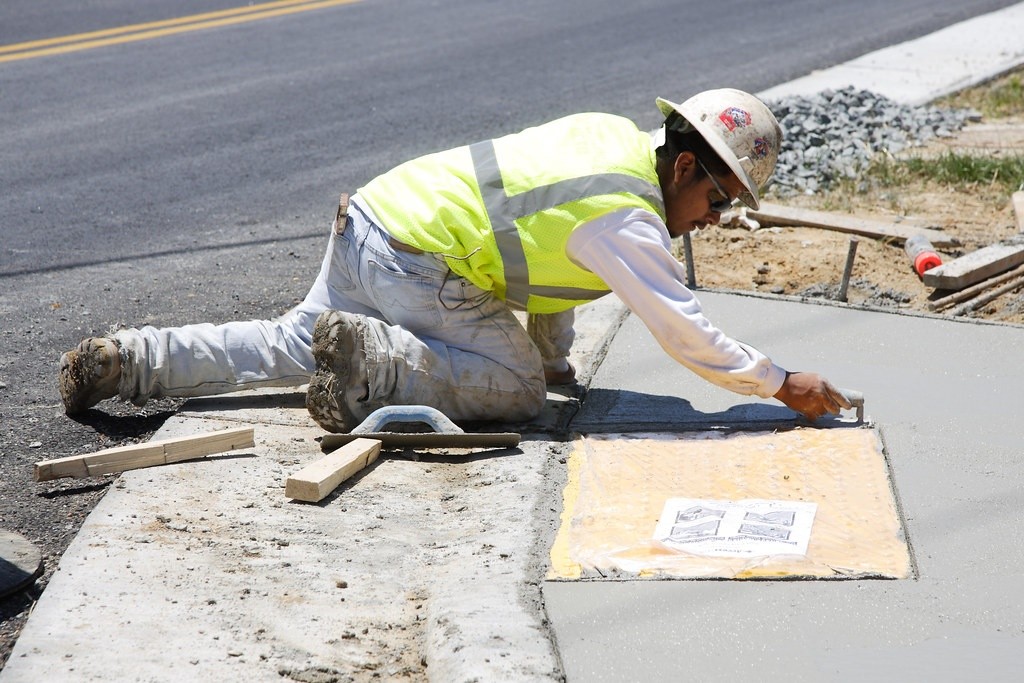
[904,235,942,276]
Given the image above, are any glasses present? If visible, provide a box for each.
[697,157,733,213]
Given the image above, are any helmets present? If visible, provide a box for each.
[656,88,783,210]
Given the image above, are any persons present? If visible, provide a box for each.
[59,87,853,435]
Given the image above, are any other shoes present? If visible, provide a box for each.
[59,337,122,415]
[305,309,368,434]
